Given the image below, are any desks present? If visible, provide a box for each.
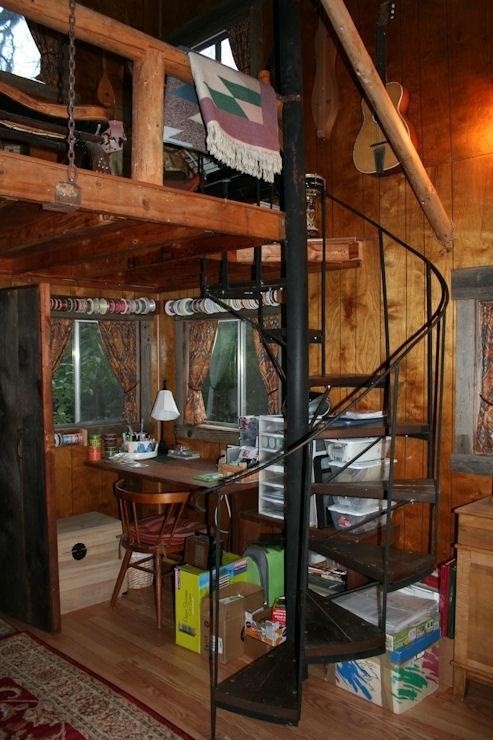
[83,456,284,558]
[236,509,400,589]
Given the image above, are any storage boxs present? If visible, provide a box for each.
[322,437,396,533]
[174,550,249,654]
[199,580,265,665]
[333,629,441,715]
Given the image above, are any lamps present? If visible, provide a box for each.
[149,388,180,456]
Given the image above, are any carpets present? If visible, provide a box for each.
[0,617,197,740]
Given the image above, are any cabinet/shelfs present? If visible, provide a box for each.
[448,494,493,701]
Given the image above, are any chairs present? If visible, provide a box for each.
[109,478,216,630]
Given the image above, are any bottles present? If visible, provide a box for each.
[180,449,193,457]
[87,433,116,461]
